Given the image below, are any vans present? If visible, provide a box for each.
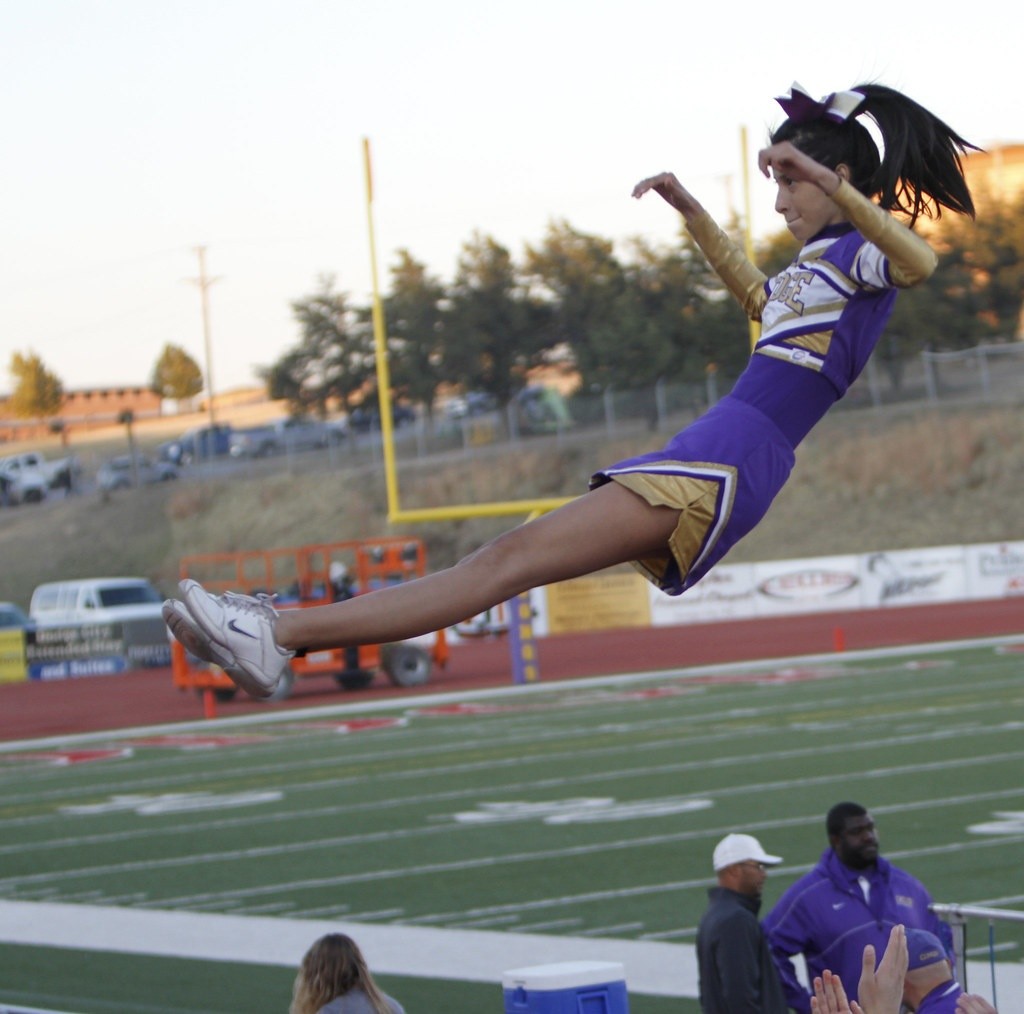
[29,577,166,626]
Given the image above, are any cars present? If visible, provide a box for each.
[0,600,32,632]
[156,397,420,465]
[93,452,183,493]
[442,378,529,420]
[0,446,82,507]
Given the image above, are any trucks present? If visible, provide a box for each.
[166,535,450,703]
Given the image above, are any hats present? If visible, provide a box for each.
[904,927,947,971]
[713,833,784,872]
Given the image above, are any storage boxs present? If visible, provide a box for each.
[501,960,630,1014]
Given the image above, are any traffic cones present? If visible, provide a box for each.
[200,687,219,719]
[832,627,846,651]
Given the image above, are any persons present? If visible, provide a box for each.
[289,932,405,1014]
[695,833,789,1014]
[160,81,978,700]
[808,924,1001,1014]
[758,802,956,1014]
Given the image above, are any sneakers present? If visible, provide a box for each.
[162,579,296,698]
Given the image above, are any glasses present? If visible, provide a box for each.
[743,862,764,872]
